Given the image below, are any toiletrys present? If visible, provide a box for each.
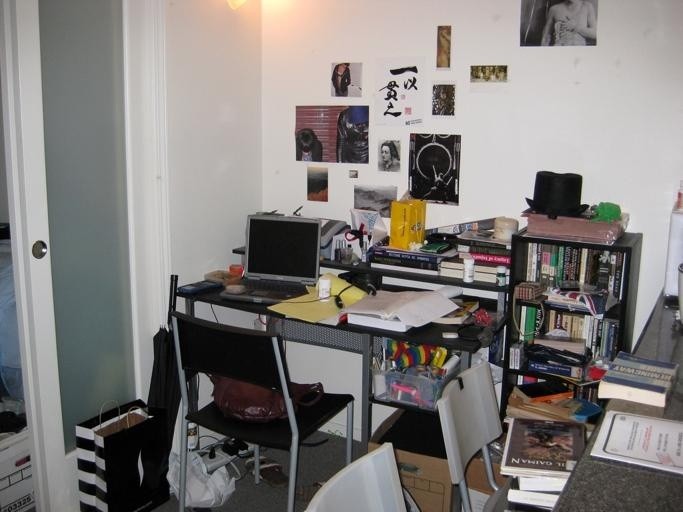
[335,240,341,263]
[340,240,347,264]
[346,243,353,265]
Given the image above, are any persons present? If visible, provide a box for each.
[379,141,400,171]
[540,0,597,46]
[332,62,351,96]
[296,128,322,162]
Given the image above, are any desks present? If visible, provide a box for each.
[552,288,683,512]
[176,264,505,444]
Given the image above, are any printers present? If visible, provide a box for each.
[310,217,351,260]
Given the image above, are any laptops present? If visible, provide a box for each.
[219,214,322,303]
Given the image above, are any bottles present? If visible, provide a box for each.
[342,239,346,249]
[463,259,474,283]
[335,240,340,263]
[496,266,506,288]
[339,241,342,249]
[188,422,199,455]
[225,264,246,295]
[319,279,331,302]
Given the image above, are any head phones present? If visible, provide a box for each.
[334,280,377,309]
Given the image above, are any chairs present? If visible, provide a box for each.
[437,362,552,512]
[304,442,421,512]
[171,311,355,512]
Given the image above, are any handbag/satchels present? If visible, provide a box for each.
[514,282,542,300]
[389,199,426,250]
[209,375,324,424]
[75,399,169,512]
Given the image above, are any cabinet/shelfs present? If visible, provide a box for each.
[500,227,643,433]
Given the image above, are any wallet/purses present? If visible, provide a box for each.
[419,243,450,254]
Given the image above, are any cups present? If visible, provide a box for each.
[491,218,519,245]
[341,247,353,265]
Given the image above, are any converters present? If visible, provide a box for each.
[222,439,239,457]
[234,438,248,451]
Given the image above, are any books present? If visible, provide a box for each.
[500,418,587,512]
[432,301,480,325]
[347,313,412,333]
[506,373,603,424]
[343,289,460,328]
[509,306,620,383]
[370,218,511,285]
[597,350,679,408]
[527,243,627,302]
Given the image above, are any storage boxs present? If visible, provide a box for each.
[368,409,462,512]
[462,455,517,512]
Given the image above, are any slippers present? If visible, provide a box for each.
[308,481,326,504]
[245,456,289,488]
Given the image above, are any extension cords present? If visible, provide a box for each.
[201,436,255,474]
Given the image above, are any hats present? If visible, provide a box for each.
[526,171,589,216]
